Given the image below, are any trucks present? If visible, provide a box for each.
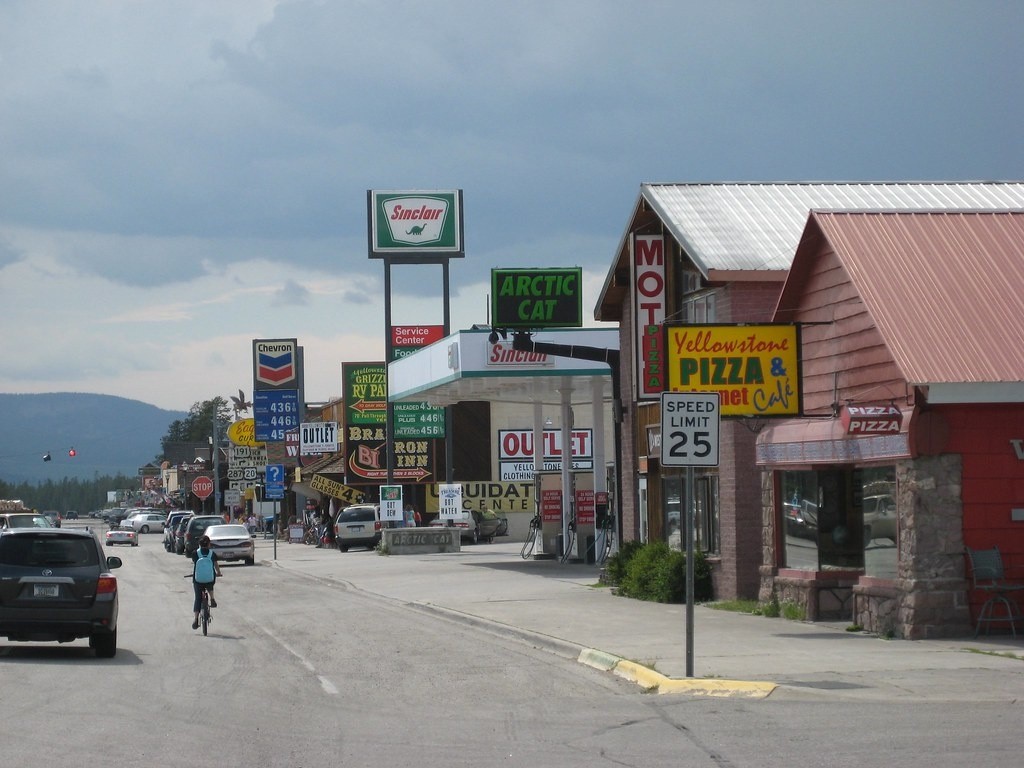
[862,494,899,549]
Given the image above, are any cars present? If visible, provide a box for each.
[430,509,501,545]
[784,502,818,539]
[105,524,139,547]
[121,514,167,535]
[42,511,62,529]
[164,515,184,552]
[173,516,195,554]
[0,513,52,533]
[89,506,157,529]
[203,523,256,567]
[66,511,79,520]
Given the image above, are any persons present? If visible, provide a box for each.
[222,511,258,538]
[191,535,221,629]
[481,505,497,543]
[397,504,422,527]
[286,512,335,548]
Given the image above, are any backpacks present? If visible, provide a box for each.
[195,548,214,583]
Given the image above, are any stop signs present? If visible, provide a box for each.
[192,475,214,501]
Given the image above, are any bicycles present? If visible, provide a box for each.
[185,571,223,635]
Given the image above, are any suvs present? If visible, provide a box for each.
[161,510,195,547]
[0,526,122,658]
[335,502,389,553]
[184,515,228,558]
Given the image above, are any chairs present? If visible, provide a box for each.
[967,545,1024,639]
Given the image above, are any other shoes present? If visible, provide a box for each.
[192,623,198,629]
[211,599,217,607]
